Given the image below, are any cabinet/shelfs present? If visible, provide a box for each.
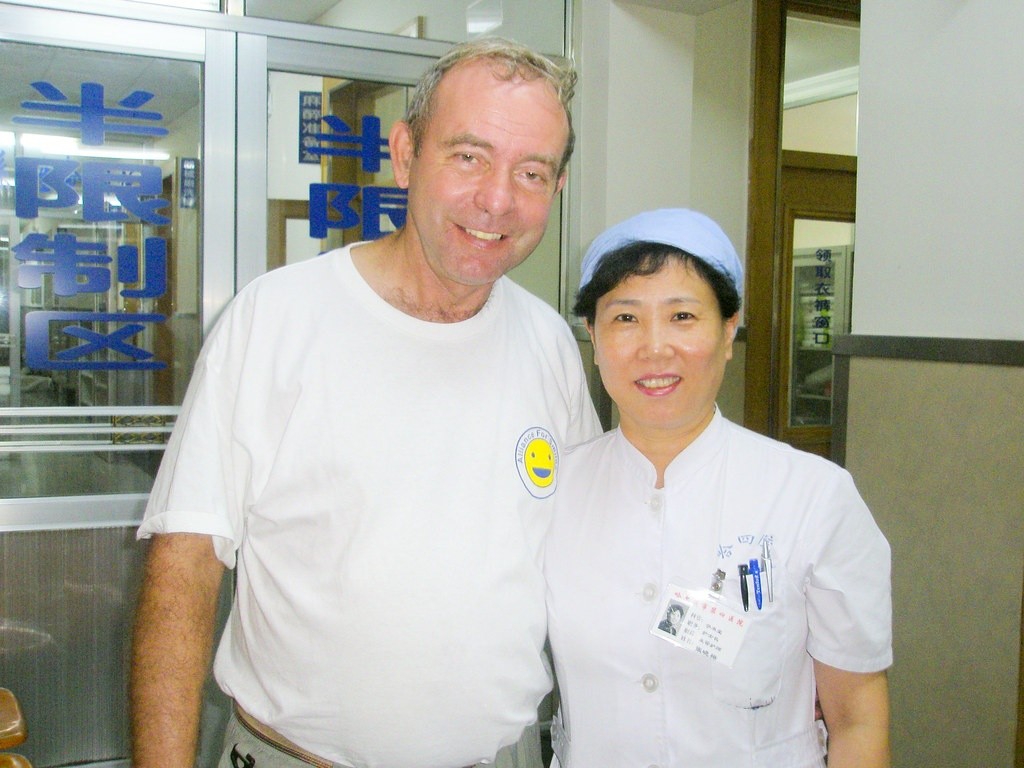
[72,213,140,433]
[788,243,856,426]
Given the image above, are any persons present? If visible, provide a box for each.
[657,603,682,635]
[544,207,894,768]
[126,35,822,768]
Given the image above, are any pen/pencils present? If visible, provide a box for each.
[736,541,776,609]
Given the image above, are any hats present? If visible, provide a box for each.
[578,209,744,345]
